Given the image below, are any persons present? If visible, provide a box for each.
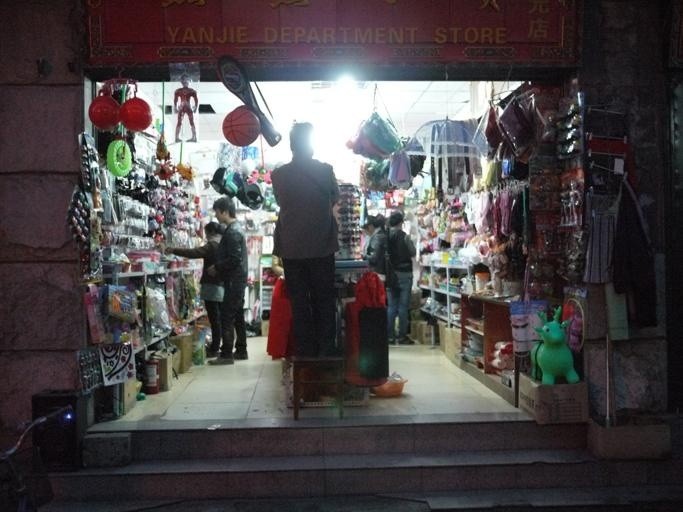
[270,121,344,358]
[355,210,418,345]
[173,74,198,143]
[205,195,249,365]
[164,221,227,358]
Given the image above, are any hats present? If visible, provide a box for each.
[210,167,264,209]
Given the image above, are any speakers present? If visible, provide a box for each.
[31,388,88,473]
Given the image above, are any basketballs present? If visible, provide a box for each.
[223,108,261,146]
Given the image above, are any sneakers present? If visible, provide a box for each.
[290,350,346,361]
[208,355,234,366]
[387,340,395,344]
[398,338,415,345]
[205,350,220,358]
[232,352,248,360]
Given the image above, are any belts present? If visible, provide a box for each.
[431,119,482,202]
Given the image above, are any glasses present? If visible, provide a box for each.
[337,185,361,256]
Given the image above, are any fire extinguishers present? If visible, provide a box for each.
[142,349,162,396]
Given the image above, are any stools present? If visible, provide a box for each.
[293,357,345,419]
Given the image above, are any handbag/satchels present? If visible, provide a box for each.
[384,256,398,288]
